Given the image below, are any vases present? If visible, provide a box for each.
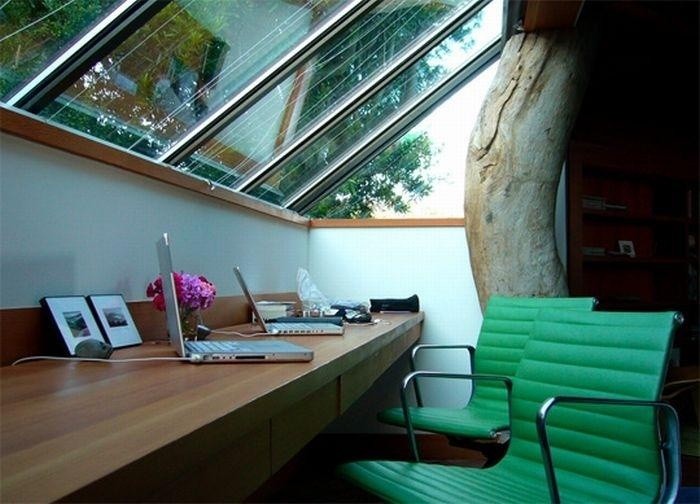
[180,311,205,340]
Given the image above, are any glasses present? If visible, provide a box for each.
[322,305,367,323]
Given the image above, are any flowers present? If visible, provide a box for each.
[146,269,218,335]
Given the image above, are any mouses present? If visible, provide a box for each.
[347,313,371,322]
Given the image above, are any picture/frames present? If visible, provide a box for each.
[38,292,143,356]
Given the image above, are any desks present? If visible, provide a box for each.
[1,307,425,504]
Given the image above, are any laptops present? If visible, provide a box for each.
[155,232,313,363]
[232,267,344,335]
